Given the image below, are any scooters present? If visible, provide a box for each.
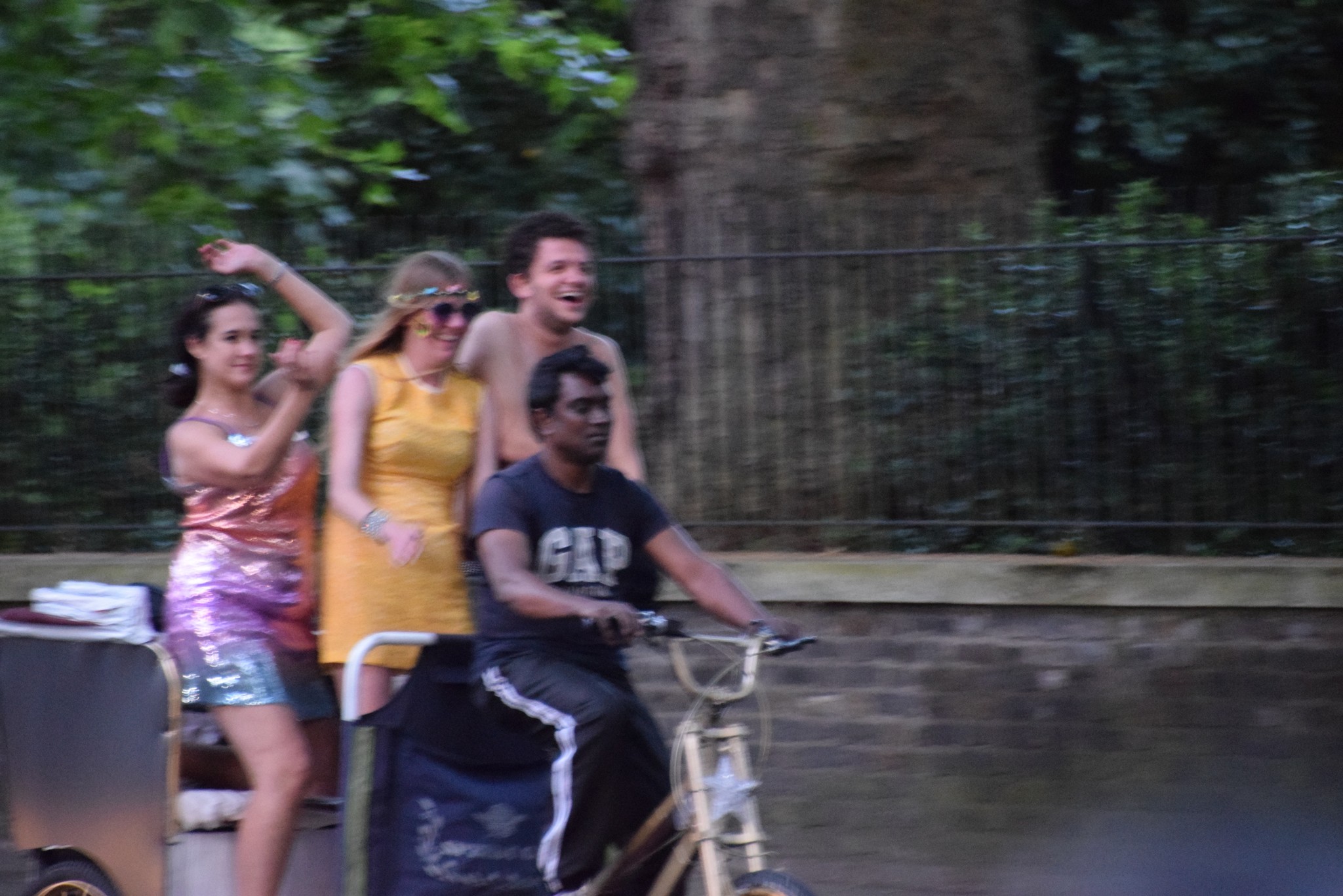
[1,581,817,896]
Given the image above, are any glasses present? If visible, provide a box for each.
[416,304,479,320]
[190,282,259,329]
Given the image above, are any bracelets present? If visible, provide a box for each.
[266,260,289,290]
[358,509,389,540]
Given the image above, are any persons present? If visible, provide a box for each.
[162,238,354,895]
[450,208,649,562]
[318,252,499,719]
[464,344,799,896]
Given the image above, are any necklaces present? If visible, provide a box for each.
[194,393,270,429]
